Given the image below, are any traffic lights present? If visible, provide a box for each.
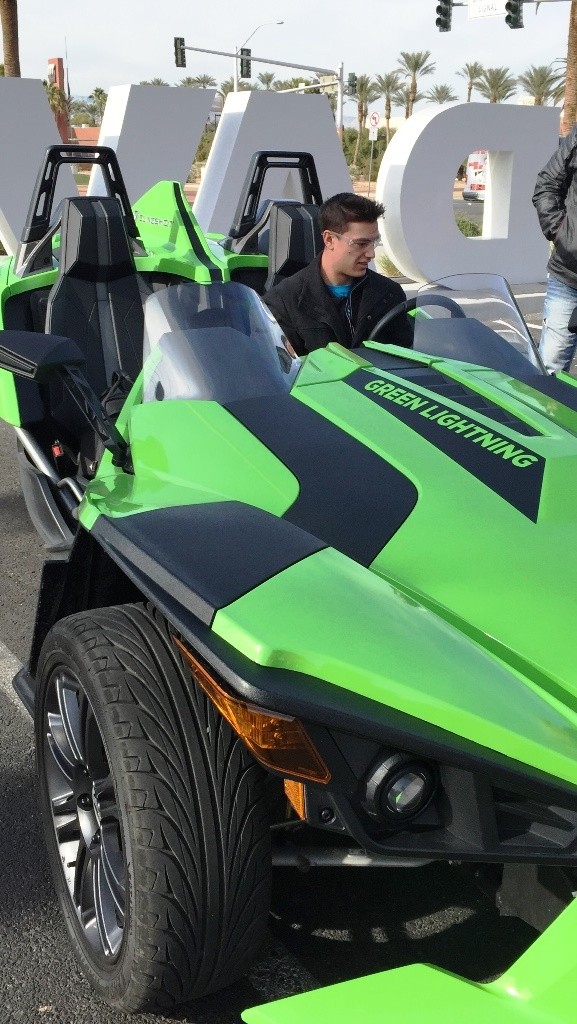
[505,0,524,29]
[348,73,357,95]
[435,0,452,32]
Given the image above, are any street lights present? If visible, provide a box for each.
[233,21,284,93]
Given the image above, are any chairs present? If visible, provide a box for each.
[263,203,325,293]
[44,196,156,480]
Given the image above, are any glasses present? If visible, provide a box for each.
[330,231,381,252]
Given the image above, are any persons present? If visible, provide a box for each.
[262,171,415,358]
[532,122,576,377]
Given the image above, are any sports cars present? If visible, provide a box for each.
[0,140,577,1024]
